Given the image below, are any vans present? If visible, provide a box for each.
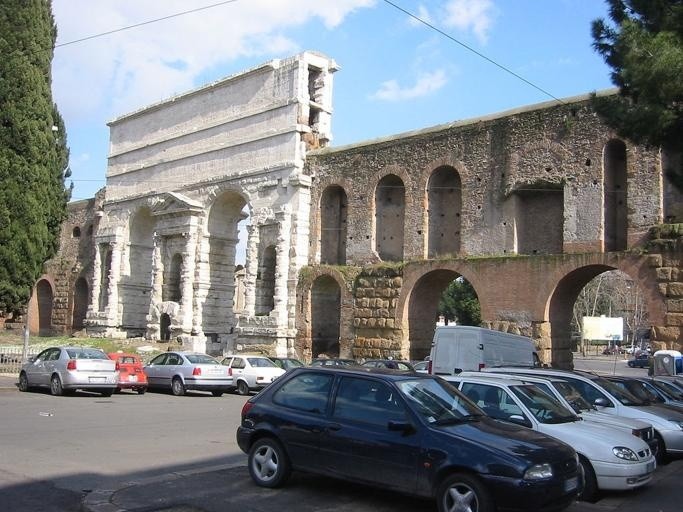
[428,325,541,375]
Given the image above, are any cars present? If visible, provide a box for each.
[390,374,657,503]
[219,354,288,397]
[309,357,359,381]
[18,345,121,397]
[141,351,233,397]
[362,360,416,373]
[236,366,587,512]
[109,352,150,395]
[459,370,656,458]
[412,361,431,373]
[482,344,683,459]
[267,357,315,385]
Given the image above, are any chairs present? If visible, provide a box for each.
[375,387,396,409]
[341,384,360,403]
[466,389,505,418]
